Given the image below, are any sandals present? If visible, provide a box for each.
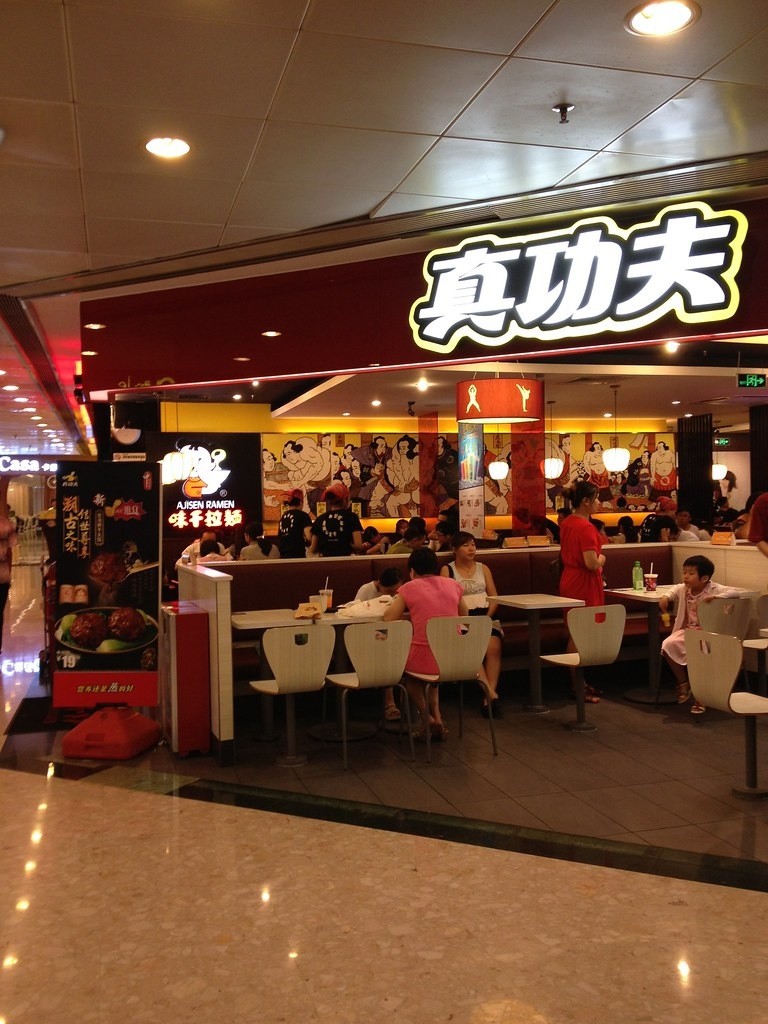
[691,701,706,715]
[675,681,692,704]
[583,684,603,703]
[383,703,401,720]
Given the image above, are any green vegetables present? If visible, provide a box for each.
[61,609,157,652]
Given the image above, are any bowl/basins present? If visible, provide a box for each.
[55,607,159,654]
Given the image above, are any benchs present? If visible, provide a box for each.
[205,546,672,665]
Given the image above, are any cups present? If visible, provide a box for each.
[310,595,327,613]
[319,590,333,608]
[644,574,658,591]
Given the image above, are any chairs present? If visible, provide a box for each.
[249,623,336,695]
[17,514,38,546]
[539,604,626,734]
[685,628,768,801]
[696,598,752,691]
[757,594,768,628]
[404,615,498,763]
[325,620,417,770]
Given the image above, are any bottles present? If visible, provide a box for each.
[633,561,644,590]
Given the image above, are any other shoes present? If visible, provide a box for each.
[481,697,505,717]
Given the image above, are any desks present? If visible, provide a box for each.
[603,585,761,704]
[487,592,586,712]
[230,607,410,768]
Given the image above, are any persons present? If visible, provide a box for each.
[748,491,768,558]
[383,547,469,743]
[353,566,407,721]
[277,495,311,558]
[181,530,235,565]
[305,484,371,558]
[0,495,56,655]
[441,531,505,718]
[515,493,764,546]
[658,556,741,714]
[359,505,459,556]
[239,521,281,561]
[559,481,606,703]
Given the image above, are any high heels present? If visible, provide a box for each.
[411,728,449,743]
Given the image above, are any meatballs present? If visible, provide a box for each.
[91,553,127,583]
[70,614,106,649]
[110,606,145,641]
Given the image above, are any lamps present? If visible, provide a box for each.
[455,359,542,424]
[488,434,509,480]
[602,384,631,472]
[623,0,702,38]
[407,401,416,417]
[545,401,564,479]
